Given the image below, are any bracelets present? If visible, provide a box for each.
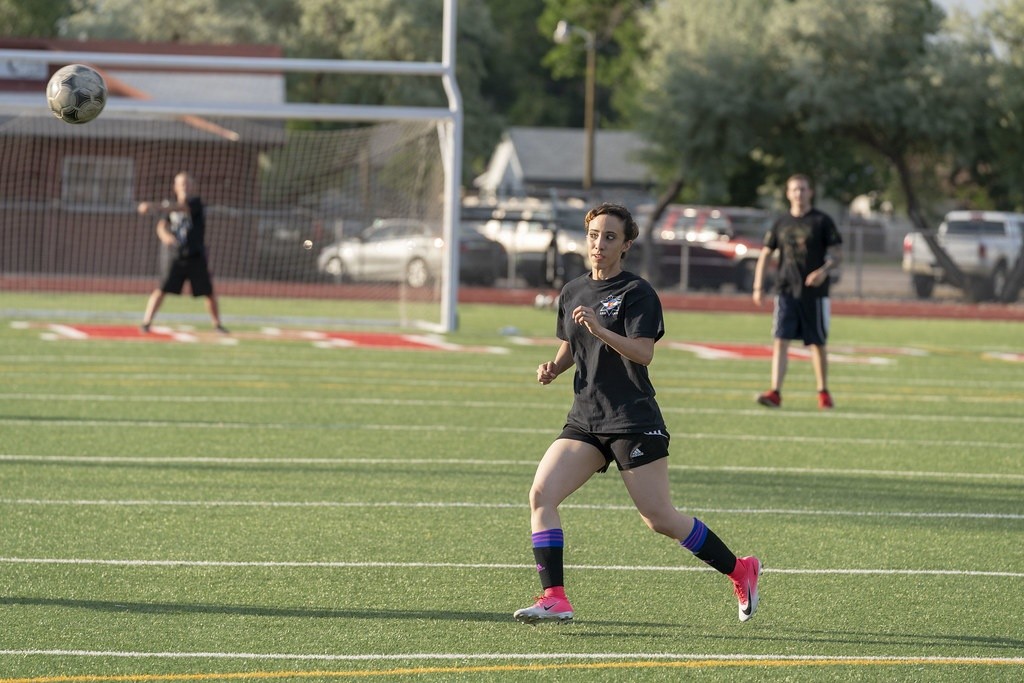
[821,265,831,276]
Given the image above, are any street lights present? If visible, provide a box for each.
[552,18,596,201]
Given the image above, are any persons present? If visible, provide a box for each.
[754,175,845,413]
[513,204,760,623]
[140,172,229,335]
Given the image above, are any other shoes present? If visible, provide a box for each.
[141,323,152,334]
[214,323,229,334]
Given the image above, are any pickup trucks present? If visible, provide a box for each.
[902,209,1024,301]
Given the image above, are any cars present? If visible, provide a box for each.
[316,217,510,291]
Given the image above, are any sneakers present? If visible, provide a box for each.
[818,390,833,409]
[513,593,573,625]
[756,391,780,406]
[734,556,762,622]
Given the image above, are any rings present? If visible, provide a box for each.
[582,310,585,315]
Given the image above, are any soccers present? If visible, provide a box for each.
[46,63,108,126]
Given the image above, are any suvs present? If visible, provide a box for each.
[632,202,785,296]
[481,194,598,290]
